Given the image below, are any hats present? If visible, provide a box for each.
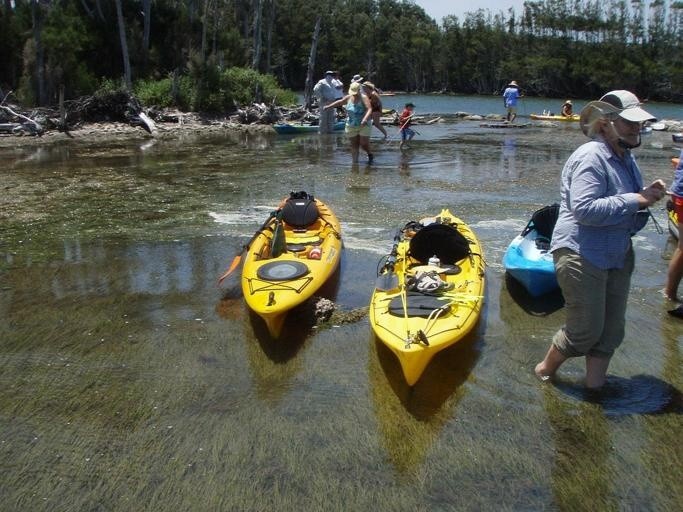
[351,74,363,83]
[565,99,572,106]
[348,82,360,95]
[507,80,518,87]
[579,89,657,138]
[362,81,375,89]
[405,103,416,107]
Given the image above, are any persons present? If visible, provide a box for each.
[312,68,388,164]
[531,88,666,393]
[661,144,683,320]
[399,101,416,148]
[500,79,525,123]
[560,98,573,117]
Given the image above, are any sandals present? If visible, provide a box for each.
[426,271,448,286]
[408,273,443,293]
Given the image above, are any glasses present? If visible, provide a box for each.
[611,121,641,151]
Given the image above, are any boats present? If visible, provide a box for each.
[502,205,563,299]
[530,109,579,121]
[241,190,343,334]
[271,120,346,133]
[378,92,395,97]
[370,206,486,386]
[380,108,396,114]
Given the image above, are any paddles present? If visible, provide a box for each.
[216,206,281,286]
[374,222,401,291]
